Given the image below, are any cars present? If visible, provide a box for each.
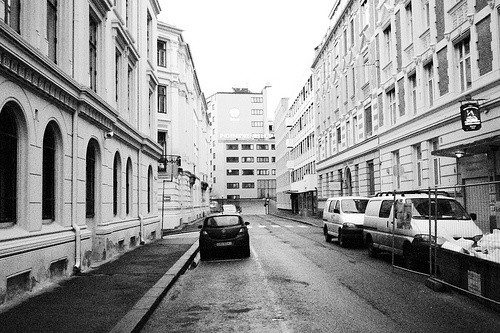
[220,205,238,213]
[197,212,251,261]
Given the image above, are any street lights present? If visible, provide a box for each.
[266,193,270,214]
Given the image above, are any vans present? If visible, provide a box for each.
[323,196,371,246]
[365,190,482,260]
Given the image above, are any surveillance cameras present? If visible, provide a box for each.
[107,131,113,138]
